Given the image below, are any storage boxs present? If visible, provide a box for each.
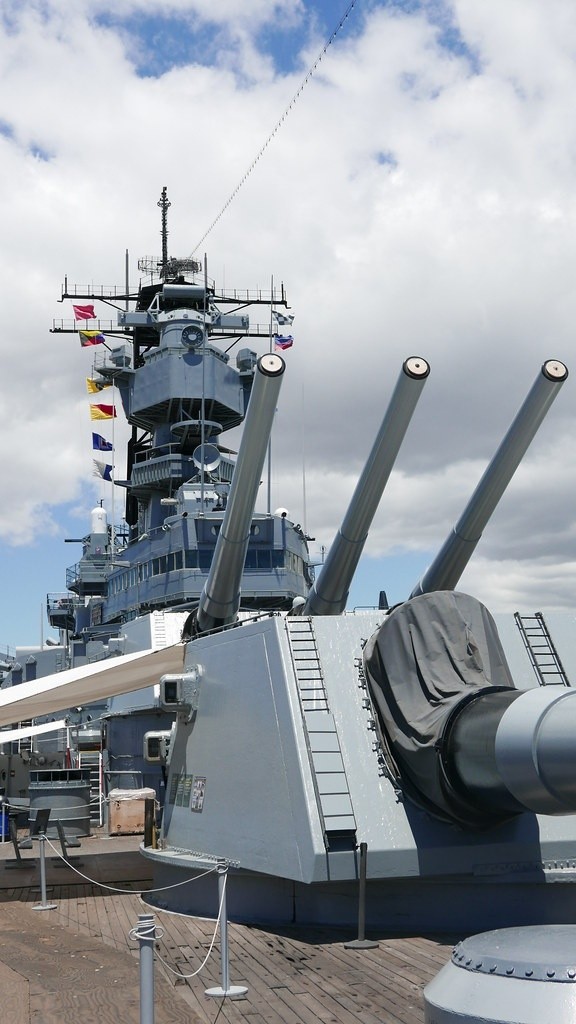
[107,787,158,834]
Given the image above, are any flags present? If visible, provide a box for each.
[93,433,112,451]
[90,404,117,420]
[79,330,105,347]
[272,311,294,326]
[275,336,294,352]
[92,458,115,482]
[86,377,110,394]
[72,304,97,321]
